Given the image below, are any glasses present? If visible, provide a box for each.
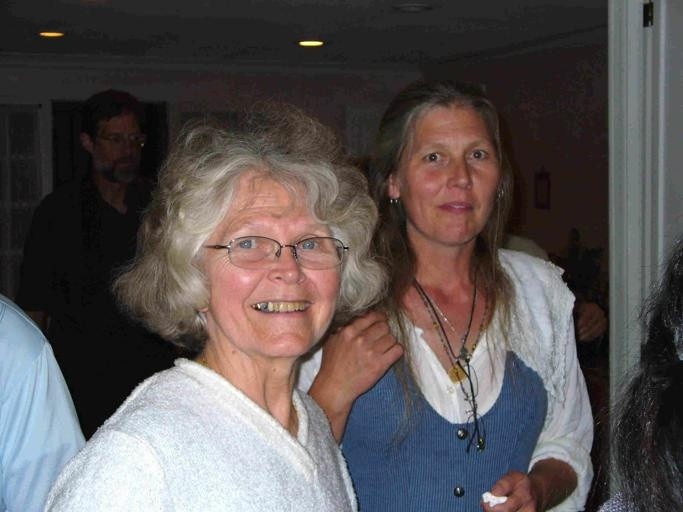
[97,133,145,146]
[205,236,348,269]
